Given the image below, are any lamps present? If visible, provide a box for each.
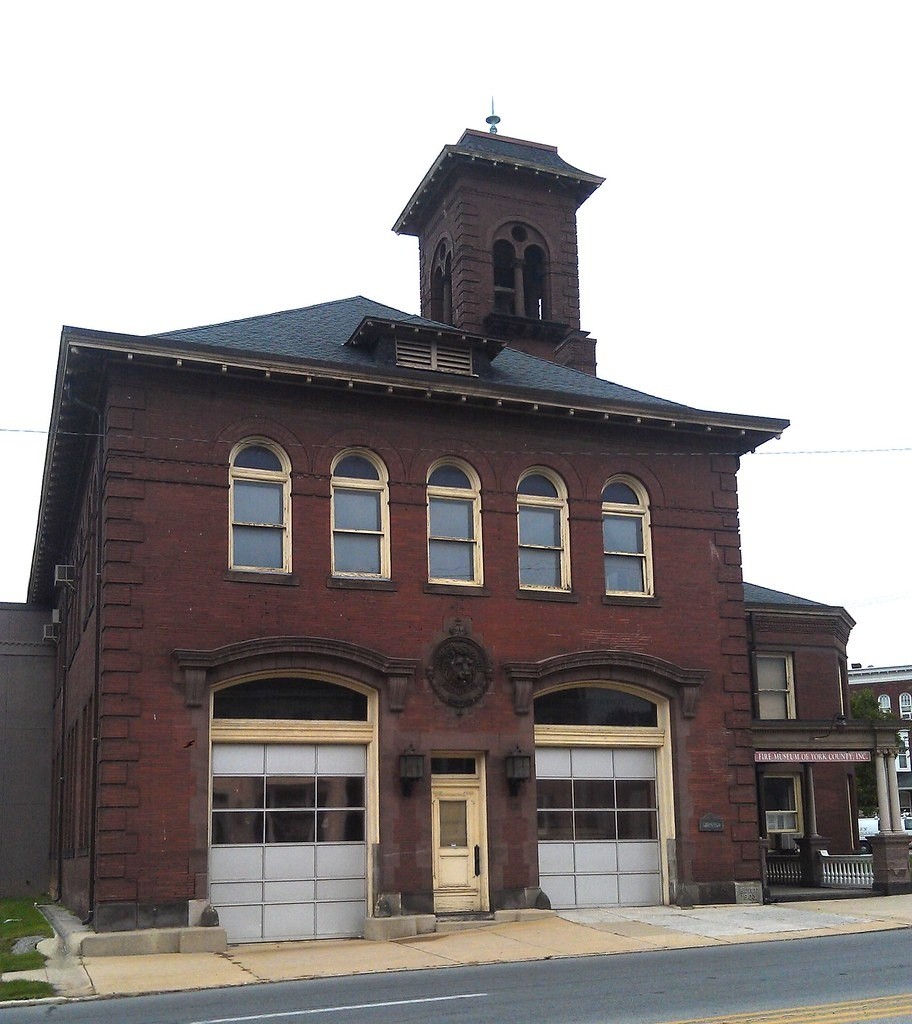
[399,743,427,797]
[506,743,532,796]
[813,712,847,740]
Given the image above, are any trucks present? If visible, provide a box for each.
[858,817,912,854]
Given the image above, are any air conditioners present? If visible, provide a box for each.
[53,565,75,591]
[42,625,57,640]
[52,609,61,624]
